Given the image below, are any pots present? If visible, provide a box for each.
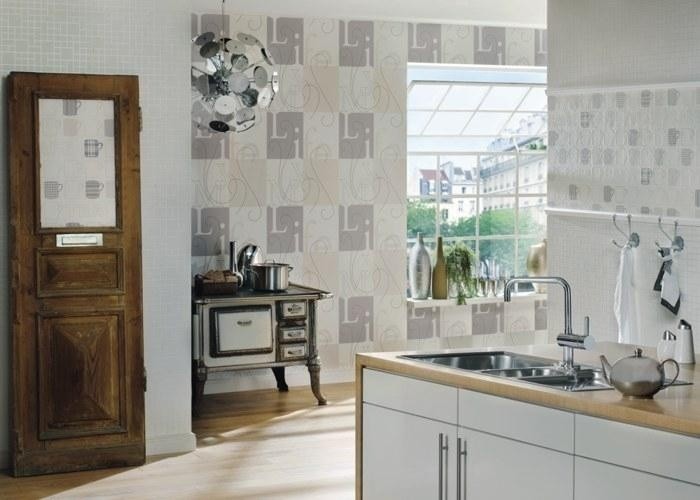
[247,263,294,292]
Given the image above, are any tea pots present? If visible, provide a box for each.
[600,348,680,400]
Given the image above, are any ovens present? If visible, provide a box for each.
[191,284,335,415]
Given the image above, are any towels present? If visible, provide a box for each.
[652,247,682,316]
[613,241,639,345]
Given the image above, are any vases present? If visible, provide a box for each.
[431,235,449,300]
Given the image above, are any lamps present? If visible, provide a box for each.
[190,0,280,135]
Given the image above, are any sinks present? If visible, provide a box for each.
[522,378,695,392]
[394,351,566,370]
[483,371,574,378]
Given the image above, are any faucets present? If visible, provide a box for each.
[503,276,590,368]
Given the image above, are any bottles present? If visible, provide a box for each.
[432,237,448,299]
[657,318,695,365]
